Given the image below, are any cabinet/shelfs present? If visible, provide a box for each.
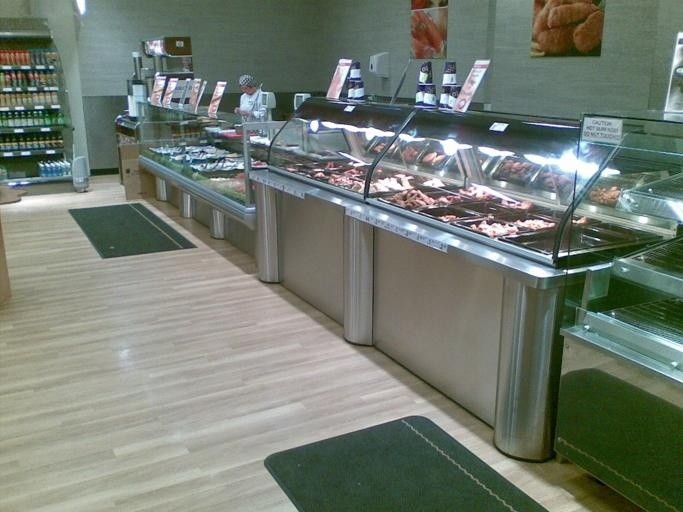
[134,100,310,259]
[1,28,74,198]
[248,95,645,463]
[551,110,683,512]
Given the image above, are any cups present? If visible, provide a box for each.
[413,61,462,111]
[347,62,366,102]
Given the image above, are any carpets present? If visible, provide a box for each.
[67,202,198,260]
[264,415,550,512]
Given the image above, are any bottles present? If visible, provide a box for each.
[0,49,71,181]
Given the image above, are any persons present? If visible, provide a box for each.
[232,73,269,123]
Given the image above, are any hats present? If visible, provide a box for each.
[238,74,259,87]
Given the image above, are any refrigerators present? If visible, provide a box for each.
[265,94,673,269]
